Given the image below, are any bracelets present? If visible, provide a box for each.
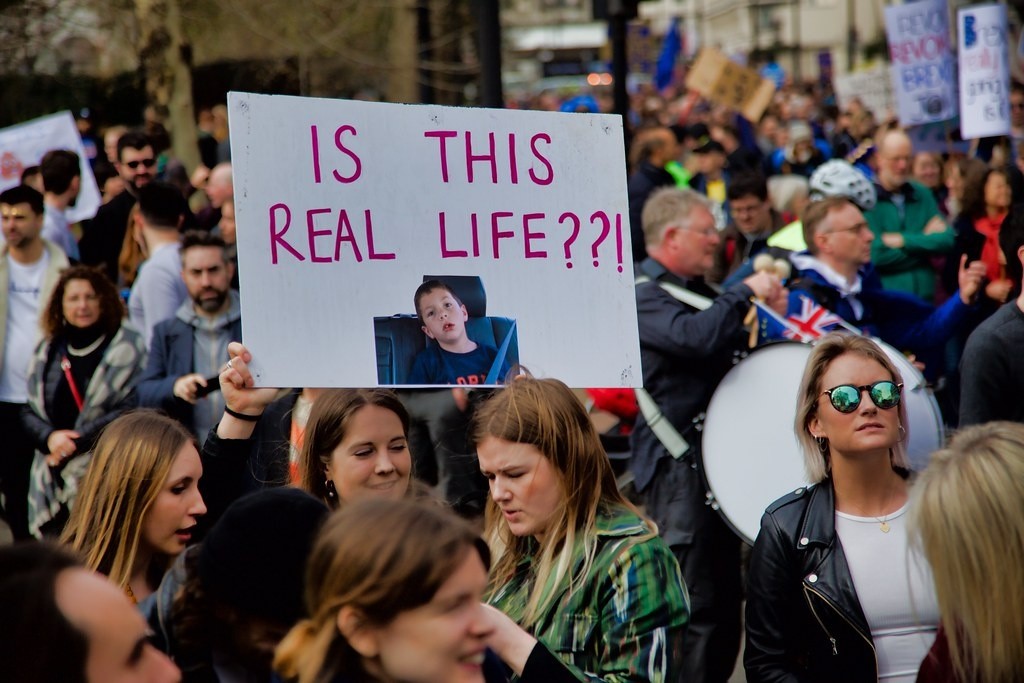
[225,404,265,421]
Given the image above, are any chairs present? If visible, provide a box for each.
[373,275,519,384]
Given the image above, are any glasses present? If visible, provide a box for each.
[121,159,156,169]
[728,202,765,213]
[677,223,721,236]
[820,219,867,236]
[814,380,904,413]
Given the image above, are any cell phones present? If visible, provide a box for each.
[195,373,219,399]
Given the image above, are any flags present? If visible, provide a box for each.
[747,290,840,345]
[651,17,688,85]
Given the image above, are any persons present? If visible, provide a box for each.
[0,81,1024,683]
[411,280,511,387]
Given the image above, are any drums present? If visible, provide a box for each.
[690,332,950,549]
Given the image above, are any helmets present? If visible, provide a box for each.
[807,158,877,211]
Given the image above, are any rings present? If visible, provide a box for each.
[60,452,66,457]
[50,461,55,466]
[227,360,232,370]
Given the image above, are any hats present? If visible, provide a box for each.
[198,487,333,627]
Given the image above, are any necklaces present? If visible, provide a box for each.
[124,581,137,603]
[830,473,899,533]
[67,331,105,356]
